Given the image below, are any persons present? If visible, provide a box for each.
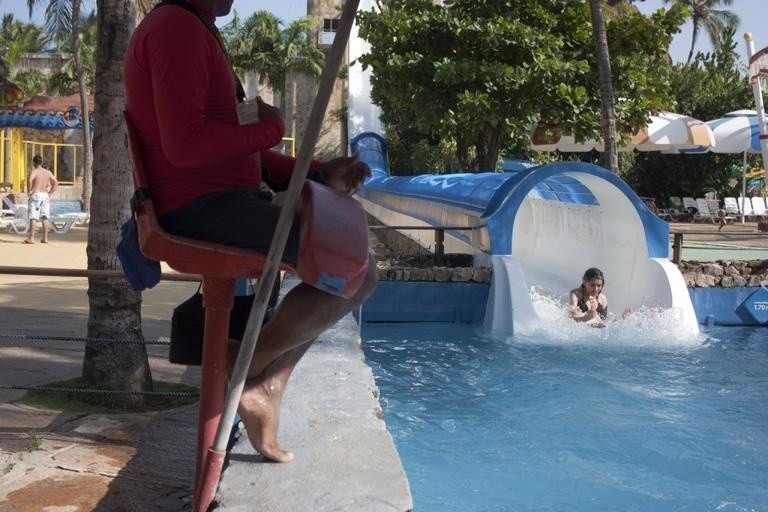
[124,0,379,463]
[717,202,727,232]
[569,268,633,330]
[24,155,57,244]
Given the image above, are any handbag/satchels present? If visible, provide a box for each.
[169,293,256,365]
[116,218,161,291]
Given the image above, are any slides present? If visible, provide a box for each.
[347,0,697,340]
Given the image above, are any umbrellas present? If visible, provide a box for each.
[531,98,768,224]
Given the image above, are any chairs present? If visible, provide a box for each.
[2,197,79,234]
[659,197,767,224]
[120,110,293,507]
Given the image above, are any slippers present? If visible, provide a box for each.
[22,240,35,244]
[40,240,49,243]
